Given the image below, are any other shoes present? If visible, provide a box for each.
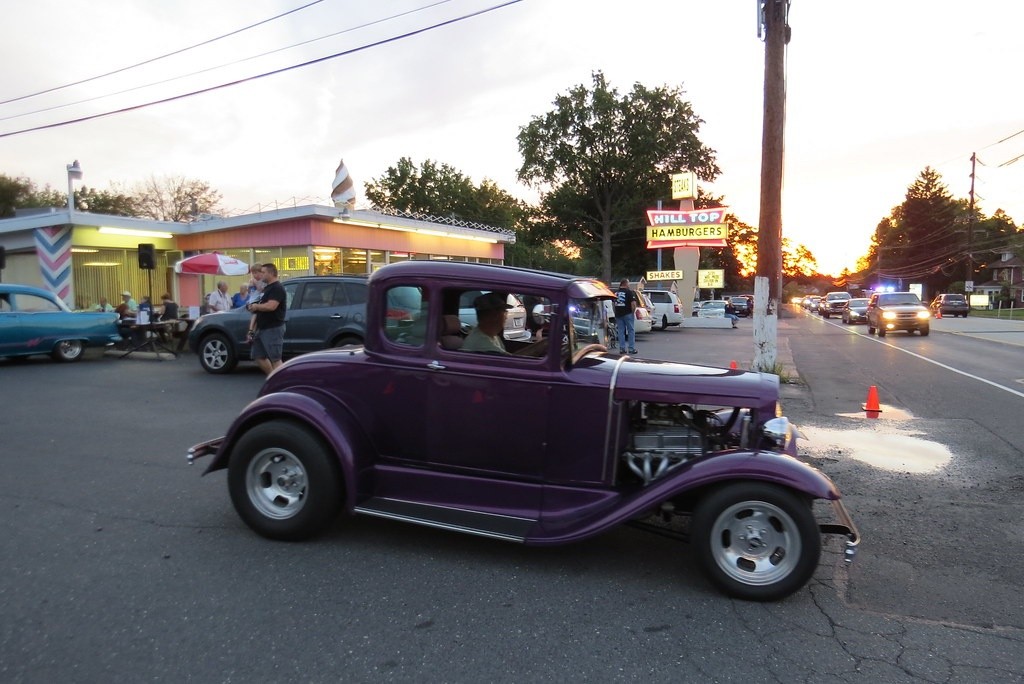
[247,329,254,340]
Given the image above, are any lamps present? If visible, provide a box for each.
[339,207,351,220]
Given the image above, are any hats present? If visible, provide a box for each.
[121,291,132,297]
[474,293,513,310]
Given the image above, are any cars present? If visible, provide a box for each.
[791,295,824,315]
[841,298,872,324]
[0,284,123,363]
[729,294,753,315]
[517,288,652,338]
[693,300,728,318]
[186,262,862,603]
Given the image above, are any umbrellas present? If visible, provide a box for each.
[175,250,250,292]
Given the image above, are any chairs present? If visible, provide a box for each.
[406,323,427,348]
[440,315,464,350]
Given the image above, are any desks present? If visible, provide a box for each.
[117,309,198,362]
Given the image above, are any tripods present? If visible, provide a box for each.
[118,270,177,361]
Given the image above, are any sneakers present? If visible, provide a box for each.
[620,348,628,354]
[629,348,638,354]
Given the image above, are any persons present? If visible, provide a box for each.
[246,263,286,375]
[160,293,177,323]
[208,281,231,313]
[724,299,739,328]
[458,293,514,355]
[139,296,154,315]
[231,284,251,309]
[248,262,267,340]
[611,279,637,354]
[95,297,112,312]
[114,303,141,351]
[121,291,138,317]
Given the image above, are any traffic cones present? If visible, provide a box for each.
[862,386,883,412]
[936,307,942,319]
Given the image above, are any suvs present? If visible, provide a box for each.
[823,292,852,319]
[866,291,930,335]
[188,275,424,375]
[459,289,531,342]
[641,289,686,329]
[930,294,970,317]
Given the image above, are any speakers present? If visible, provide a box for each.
[138,243,156,270]
